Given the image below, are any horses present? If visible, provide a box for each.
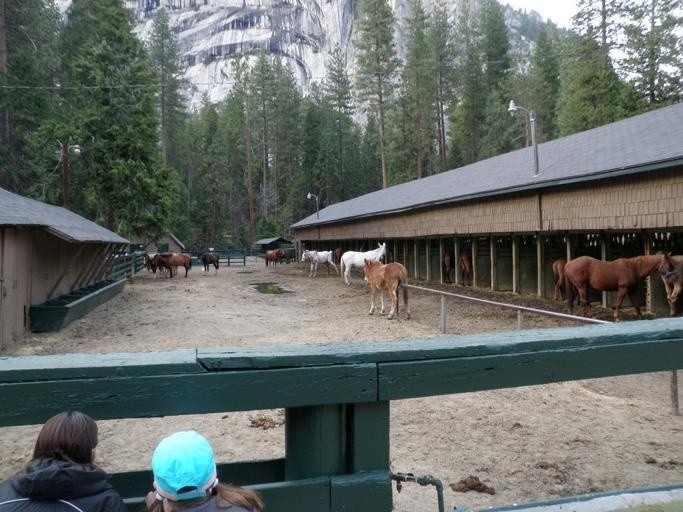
[143,252,192,279]
[364,258,411,320]
[301,249,340,278]
[335,248,345,265]
[552,257,577,301]
[661,255,683,316]
[339,241,386,287]
[201,252,219,272]
[565,250,681,321]
[444,253,473,287]
[265,248,288,268]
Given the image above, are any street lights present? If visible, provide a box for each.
[507,100,539,174]
[63,142,81,210]
[307,191,319,219]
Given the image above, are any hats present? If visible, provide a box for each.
[151,430,218,501]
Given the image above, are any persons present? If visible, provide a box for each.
[0,410,130,512]
[137,430,265,511]
